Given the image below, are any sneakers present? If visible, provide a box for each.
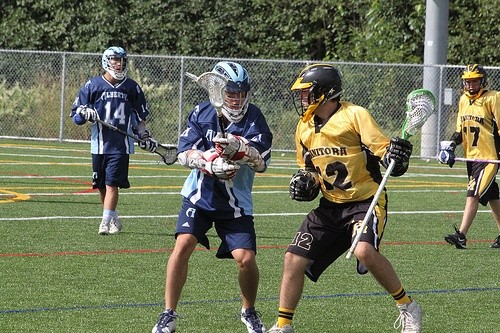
[265,322,294,333]
[109,214,121,234]
[151,309,176,333]
[445,226,467,249]
[394,295,422,333]
[492,235,500,248]
[240,307,266,333]
[98,218,109,234]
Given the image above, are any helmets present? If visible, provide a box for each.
[461,64,488,100]
[102,46,128,80]
[291,63,344,123]
[211,61,251,123]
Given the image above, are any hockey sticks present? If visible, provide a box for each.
[195,71,233,165]
[345,88,436,260]
[454,157,500,164]
[94,118,179,165]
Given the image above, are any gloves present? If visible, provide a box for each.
[437,140,456,168]
[215,135,251,162]
[289,173,315,201]
[386,137,413,166]
[76,105,97,122]
[205,153,241,180]
[135,128,158,153]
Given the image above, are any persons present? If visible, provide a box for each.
[265,64,423,333]
[437,64,500,249]
[152,61,273,333]
[69,46,158,235]
